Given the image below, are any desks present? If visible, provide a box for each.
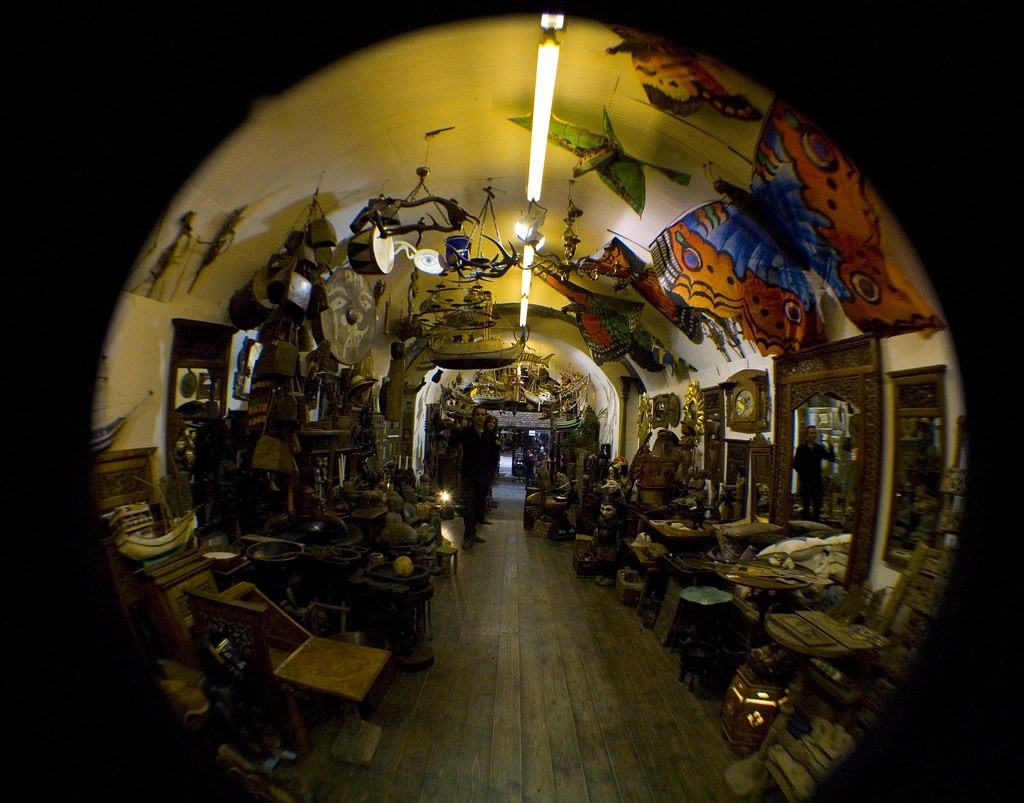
[630,509,715,540]
[714,558,816,627]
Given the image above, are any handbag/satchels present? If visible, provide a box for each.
[250,434,295,474]
[228,202,337,432]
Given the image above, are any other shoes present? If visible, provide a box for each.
[473,533,486,543]
[481,521,493,525]
[463,536,475,549]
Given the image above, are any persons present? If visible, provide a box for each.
[593,501,623,586]
[448,405,503,551]
[793,425,836,522]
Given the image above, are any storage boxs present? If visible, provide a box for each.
[572,552,597,578]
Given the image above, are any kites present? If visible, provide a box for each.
[535,237,700,377]
[647,96,945,357]
[509,113,691,221]
[601,22,763,122]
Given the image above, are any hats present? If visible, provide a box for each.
[180,372,197,398]
[349,375,374,394]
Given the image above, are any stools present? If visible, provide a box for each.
[437,547,459,576]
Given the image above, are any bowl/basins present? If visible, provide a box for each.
[305,521,342,537]
[202,551,238,570]
[246,541,304,578]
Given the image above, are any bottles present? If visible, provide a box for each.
[248,501,288,537]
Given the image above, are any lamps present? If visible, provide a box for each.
[515,198,548,251]
